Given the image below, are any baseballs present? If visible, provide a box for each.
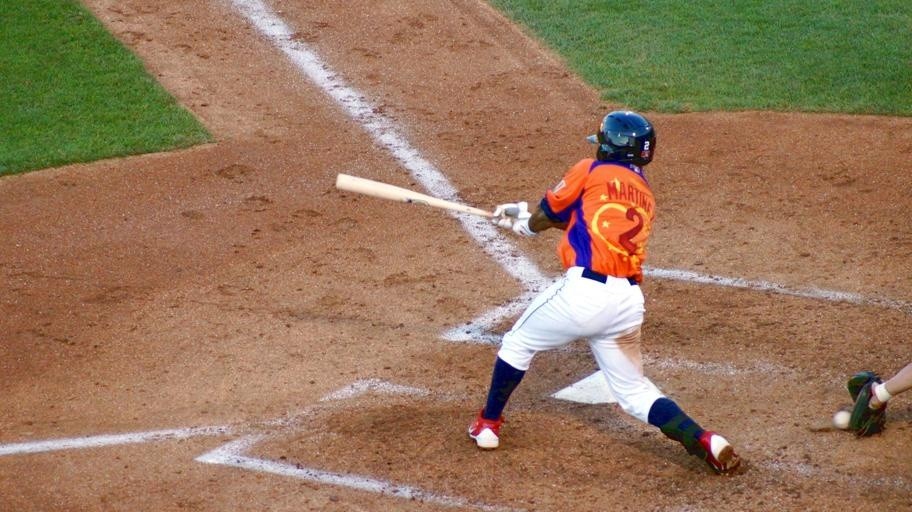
[834,411,851,429]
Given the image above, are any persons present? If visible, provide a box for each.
[466,109,747,478]
[846,361,911,440]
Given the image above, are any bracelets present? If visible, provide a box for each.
[874,382,892,403]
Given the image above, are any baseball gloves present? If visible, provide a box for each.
[848,370,886,437]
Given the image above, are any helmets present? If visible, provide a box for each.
[586,110,655,166]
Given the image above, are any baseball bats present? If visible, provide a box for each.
[335,173,519,223]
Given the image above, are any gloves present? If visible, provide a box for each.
[491,200,537,238]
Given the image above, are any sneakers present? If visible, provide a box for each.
[849,377,887,436]
[468,408,504,450]
[699,431,740,475]
